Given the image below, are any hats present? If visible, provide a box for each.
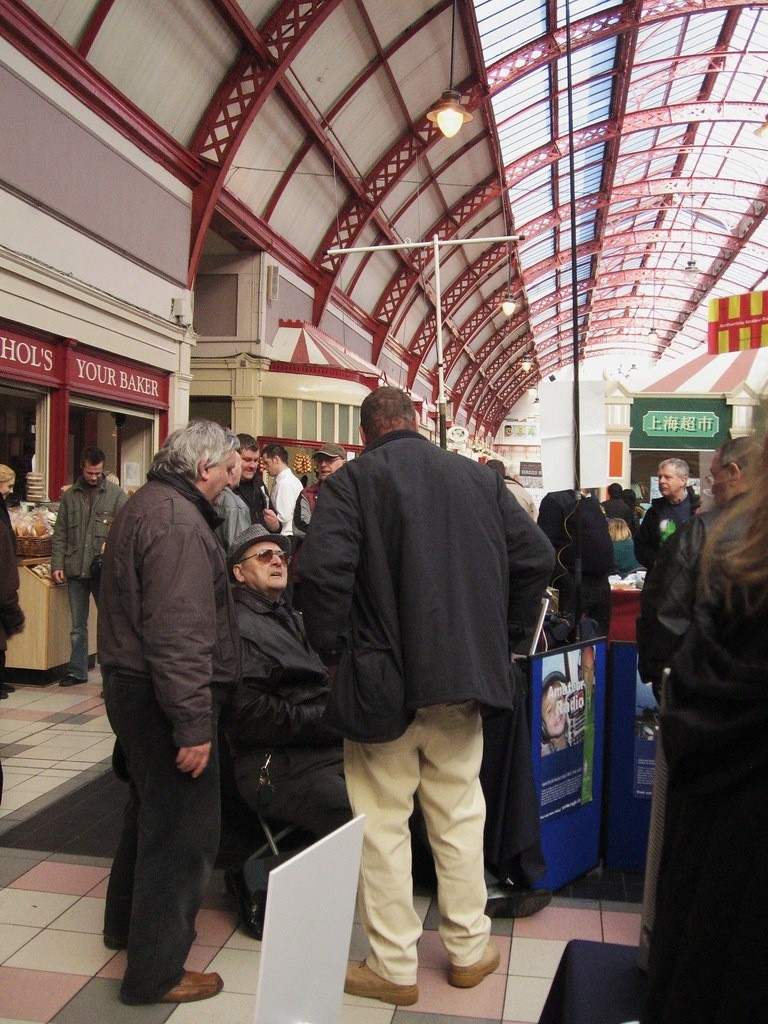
[226,523,292,584]
[311,443,347,459]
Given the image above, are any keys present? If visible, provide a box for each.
[255,776,270,793]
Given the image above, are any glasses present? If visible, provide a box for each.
[704,462,742,485]
[317,457,341,463]
[238,549,290,567]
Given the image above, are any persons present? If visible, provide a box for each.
[485,459,646,645]
[211,432,347,552]
[217,524,553,920]
[50,447,128,698]
[301,390,556,1006]
[0,463,26,701]
[638,437,768,1024]
[104,418,245,1001]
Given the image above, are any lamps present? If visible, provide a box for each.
[626,318,641,373]
[682,193,703,279]
[753,112,768,137]
[519,296,535,371]
[647,268,659,341]
[527,365,536,395]
[534,373,540,407]
[501,220,518,315]
[427,0,473,140]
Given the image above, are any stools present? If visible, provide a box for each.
[220,814,300,897]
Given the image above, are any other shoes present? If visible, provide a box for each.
[59,676,87,686]
[486,882,552,918]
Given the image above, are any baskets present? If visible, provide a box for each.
[15,536,53,556]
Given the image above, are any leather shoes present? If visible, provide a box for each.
[224,859,267,941]
[119,969,223,1007]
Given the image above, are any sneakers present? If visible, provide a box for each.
[449,942,500,989]
[344,959,420,1006]
